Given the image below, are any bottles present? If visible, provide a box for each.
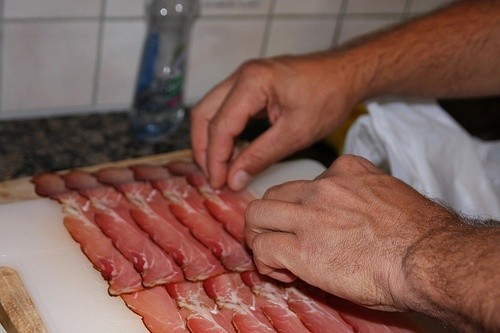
[130,0,198,145]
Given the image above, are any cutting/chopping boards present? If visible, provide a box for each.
[1,140,251,332]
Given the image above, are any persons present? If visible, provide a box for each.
[188,0,499,333]
